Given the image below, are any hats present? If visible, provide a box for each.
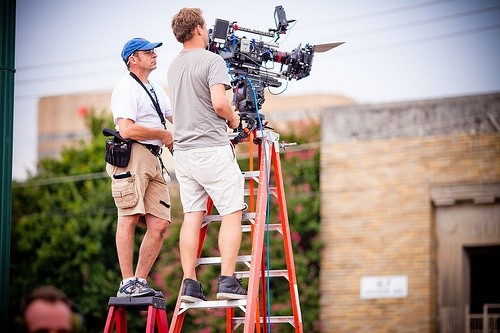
[121,38,162,62]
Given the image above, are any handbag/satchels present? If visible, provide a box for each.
[103,129,132,167]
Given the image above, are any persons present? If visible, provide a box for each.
[164,7,248,304]
[21,286,73,333]
[105,38,174,298]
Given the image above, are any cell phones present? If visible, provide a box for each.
[214,18,229,43]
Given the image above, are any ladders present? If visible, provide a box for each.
[103,296,170,333]
[169,129,305,333]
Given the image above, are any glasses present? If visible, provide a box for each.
[132,50,155,56]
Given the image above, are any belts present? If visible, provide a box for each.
[133,140,161,157]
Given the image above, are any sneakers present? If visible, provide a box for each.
[217,277,247,300]
[117,279,164,298]
[181,278,207,303]
